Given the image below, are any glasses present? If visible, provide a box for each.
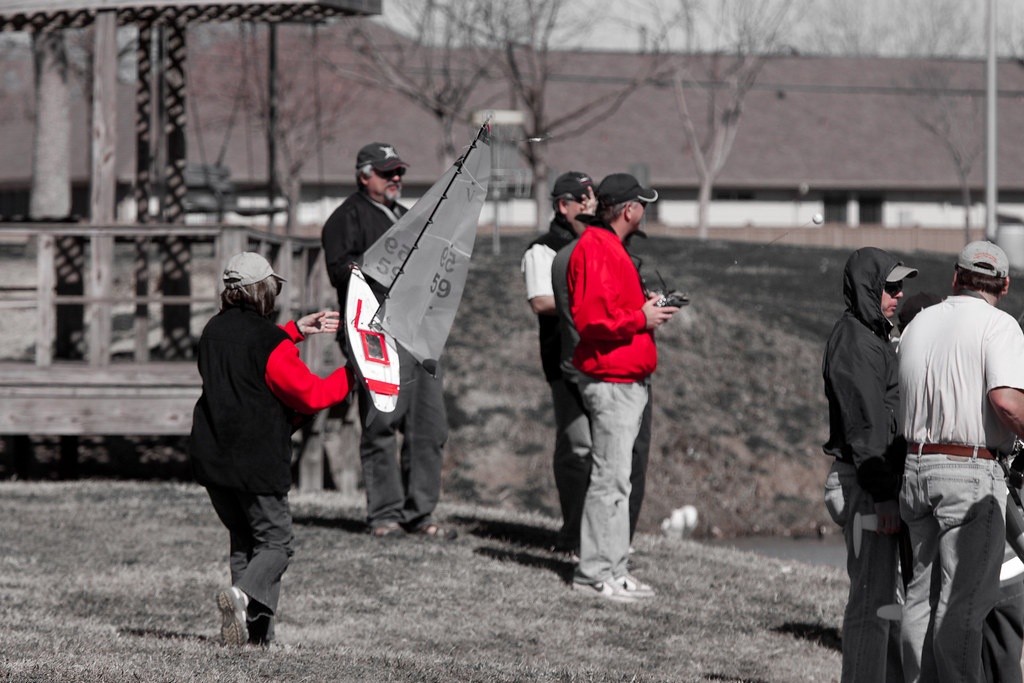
[565,197,582,203]
[883,280,903,294]
[629,198,647,207]
[368,167,406,177]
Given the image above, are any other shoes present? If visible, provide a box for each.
[414,520,458,540]
[367,521,405,538]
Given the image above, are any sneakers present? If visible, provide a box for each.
[573,575,637,604]
[218,586,250,645]
[613,570,654,597]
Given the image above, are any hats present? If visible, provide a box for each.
[957,241,1009,278]
[223,252,285,289]
[356,142,410,171]
[597,173,658,203]
[885,264,919,282]
[553,171,598,197]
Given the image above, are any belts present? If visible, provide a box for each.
[909,443,997,460]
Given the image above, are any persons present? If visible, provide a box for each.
[188,253,356,650]
[894,288,942,597]
[321,141,457,544]
[518,171,681,600]
[821,246,919,683]
[896,241,1024,683]
